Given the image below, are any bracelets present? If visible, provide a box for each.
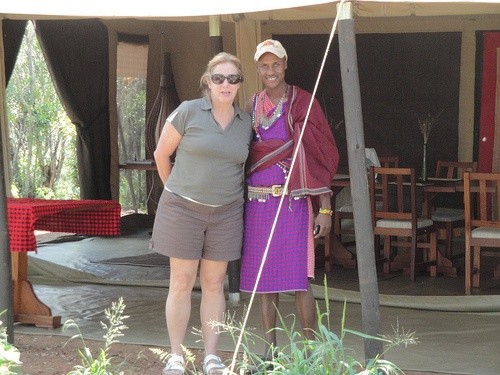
[318,208,334,216]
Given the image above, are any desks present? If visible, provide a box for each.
[330,173,497,278]
[115,160,175,235]
[6,196,121,328]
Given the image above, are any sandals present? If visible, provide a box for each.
[162,355,187,375]
[202,354,228,375]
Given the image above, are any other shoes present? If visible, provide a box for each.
[245,353,281,375]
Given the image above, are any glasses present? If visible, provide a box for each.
[210,74,242,85]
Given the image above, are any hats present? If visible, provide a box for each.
[254,39,288,63]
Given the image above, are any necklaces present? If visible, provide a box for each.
[259,83,289,127]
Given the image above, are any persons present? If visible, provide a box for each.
[242,39,339,375]
[149,52,253,375]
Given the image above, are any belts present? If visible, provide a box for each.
[247,185,289,197]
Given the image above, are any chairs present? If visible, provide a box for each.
[463,172,500,295]
[334,157,400,260]
[370,166,437,288]
[431,160,478,263]
